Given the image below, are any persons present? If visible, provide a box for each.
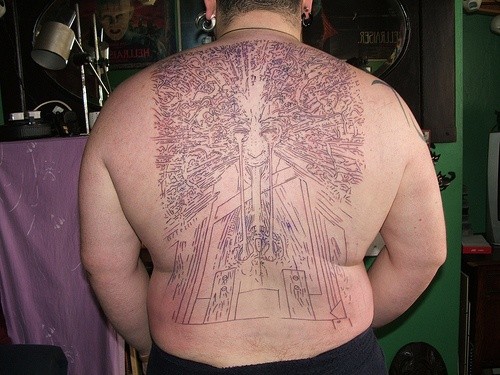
[96,0,166,65]
[76,0,449,375]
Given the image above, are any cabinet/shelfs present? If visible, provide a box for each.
[458,232,499,375]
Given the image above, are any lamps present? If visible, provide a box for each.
[31,0,109,101]
